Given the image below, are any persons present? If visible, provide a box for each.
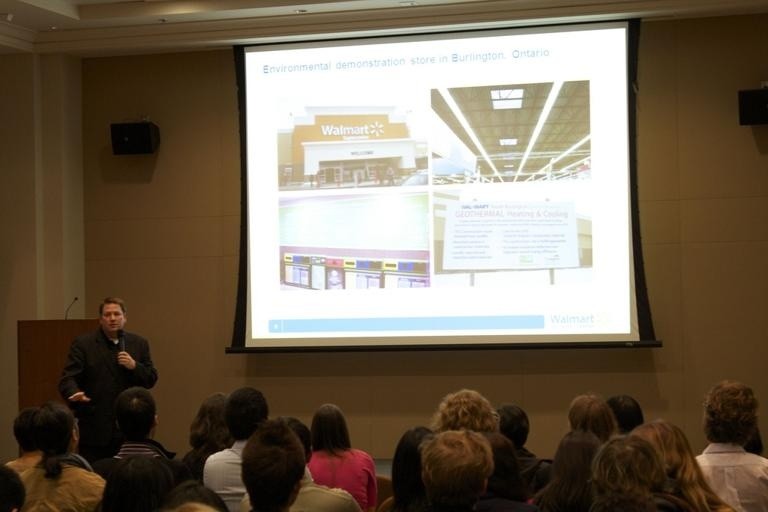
[61,297,159,459]
[0,387,378,512]
[378,382,768,512]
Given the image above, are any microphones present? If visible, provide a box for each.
[117,329,126,352]
[65,297,78,319]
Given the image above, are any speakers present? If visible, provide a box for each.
[738,88,768,126]
[111,121,160,156]
